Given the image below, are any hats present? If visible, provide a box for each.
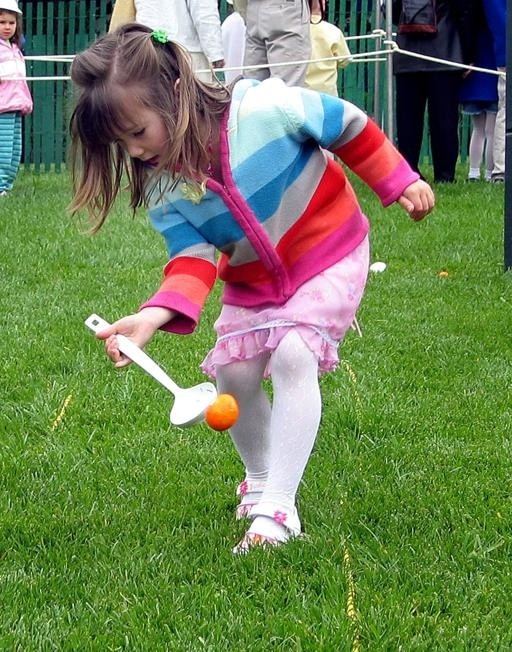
[1,1,24,14]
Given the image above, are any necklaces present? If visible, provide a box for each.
[180,116,215,205]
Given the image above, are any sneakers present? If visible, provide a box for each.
[468,178,480,184]
[491,174,504,184]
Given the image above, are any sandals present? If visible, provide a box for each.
[231,505,301,556]
[235,480,269,522]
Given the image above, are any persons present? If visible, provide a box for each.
[380,1,486,183]
[62,22,436,553]
[460,23,499,183]
[482,1,506,184]
[105,0,352,96]
[0,1,34,202]
